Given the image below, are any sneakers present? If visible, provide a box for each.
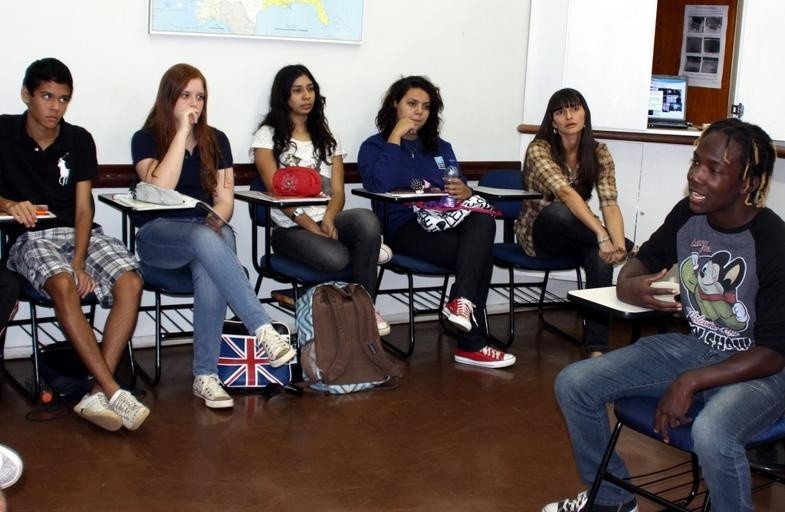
[378,243,394,266]
[192,373,234,410]
[375,308,391,337]
[109,388,151,431]
[453,344,517,369]
[72,391,123,432]
[442,294,479,334]
[254,322,296,369]
[542,489,638,511]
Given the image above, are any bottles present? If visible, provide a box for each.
[30,375,55,403]
[440,158,458,208]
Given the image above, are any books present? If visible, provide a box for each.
[112,188,237,233]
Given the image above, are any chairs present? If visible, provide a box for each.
[2,171,585,404]
[567,281,784,511]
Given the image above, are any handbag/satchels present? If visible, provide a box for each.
[217,320,292,399]
[25,338,147,423]
[272,167,322,196]
[403,176,503,233]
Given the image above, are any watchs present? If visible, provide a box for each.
[291,207,305,222]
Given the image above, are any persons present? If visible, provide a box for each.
[357,76,517,369]
[248,65,394,337]
[538,118,785,512]
[131,64,296,409]
[512,88,640,359]
[0,264,24,512]
[0,58,151,432]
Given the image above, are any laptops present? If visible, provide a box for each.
[648,74,688,129]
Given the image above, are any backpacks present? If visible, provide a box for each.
[293,280,401,393]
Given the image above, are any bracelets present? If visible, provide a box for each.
[597,237,610,244]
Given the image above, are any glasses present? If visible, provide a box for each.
[423,198,456,209]
